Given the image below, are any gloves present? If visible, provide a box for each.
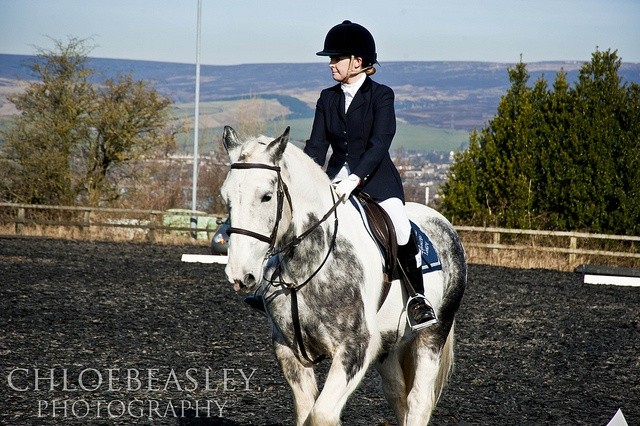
[333,174,361,201]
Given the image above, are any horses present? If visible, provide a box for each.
[219,126,469,426]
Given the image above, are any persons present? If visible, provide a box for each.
[301,19,440,331]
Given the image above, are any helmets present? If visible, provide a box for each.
[316,20,377,63]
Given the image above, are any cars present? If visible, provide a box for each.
[211,217,229,253]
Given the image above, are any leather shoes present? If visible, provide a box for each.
[407,295,436,324]
[244,295,265,312]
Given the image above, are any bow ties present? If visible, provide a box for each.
[341,85,356,97]
